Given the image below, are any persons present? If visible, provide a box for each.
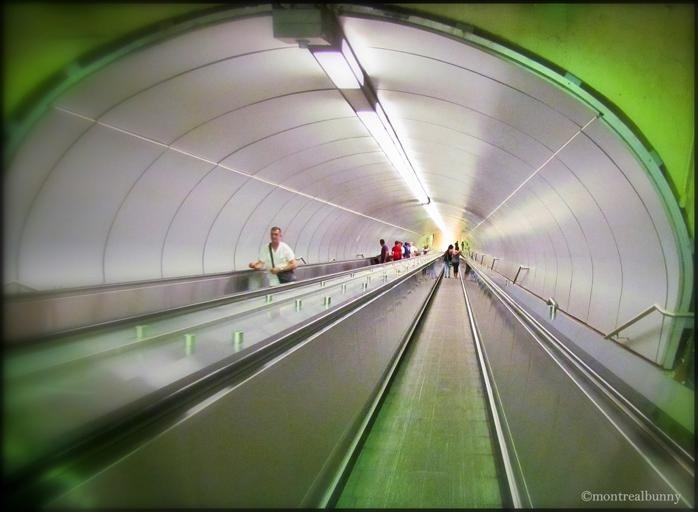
[455,241,459,250]
[461,241,464,251]
[376,237,429,262]
[443,244,454,278]
[452,247,463,278]
[250,227,297,286]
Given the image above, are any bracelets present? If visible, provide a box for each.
[280,266,284,272]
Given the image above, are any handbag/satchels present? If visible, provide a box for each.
[278,270,297,283]
[448,261,452,267]
